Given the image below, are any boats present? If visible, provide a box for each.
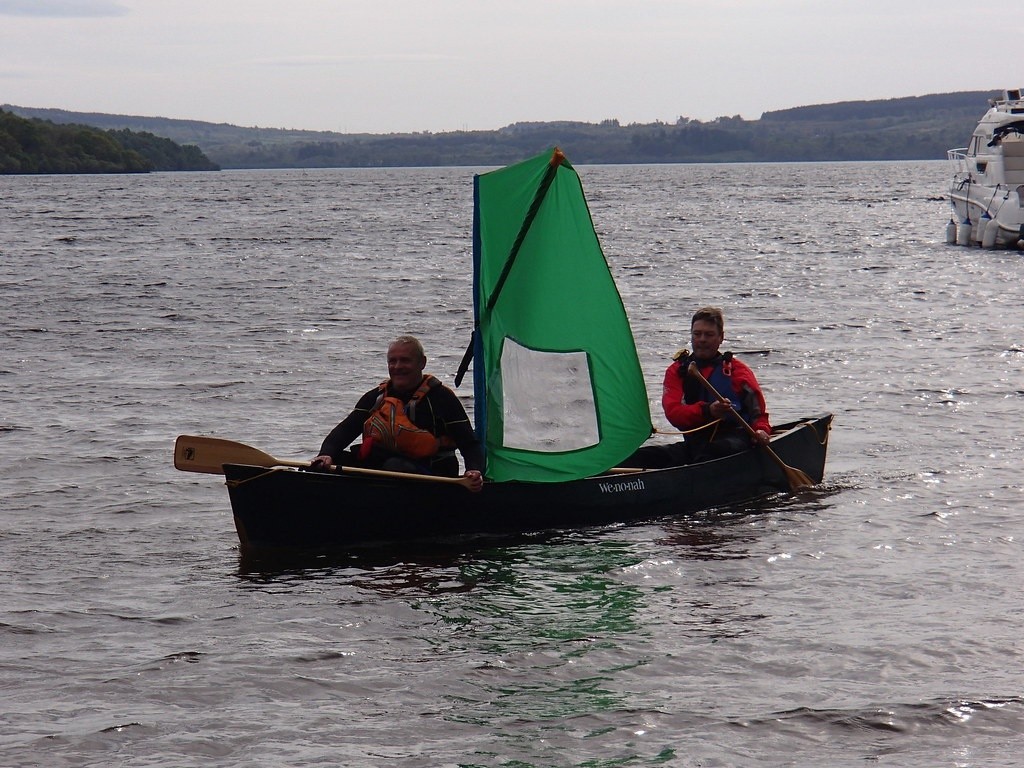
[946,87,1024,251]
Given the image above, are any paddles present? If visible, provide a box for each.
[686,361,816,489]
[173,434,476,492]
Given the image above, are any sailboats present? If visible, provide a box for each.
[221,144,833,546]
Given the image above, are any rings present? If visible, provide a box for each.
[764,436,768,440]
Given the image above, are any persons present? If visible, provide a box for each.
[311,335,484,493]
[658,307,771,468]
